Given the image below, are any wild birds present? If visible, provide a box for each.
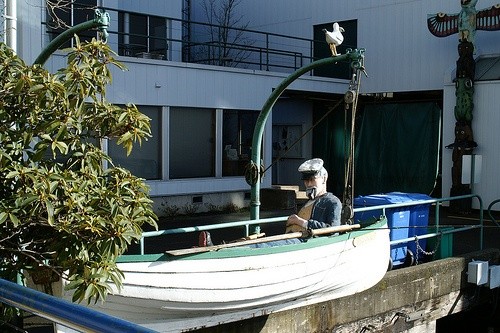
[323,22,344,56]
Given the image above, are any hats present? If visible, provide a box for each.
[298,158,324,180]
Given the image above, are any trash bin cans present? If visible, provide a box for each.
[389,192,432,267]
[354,195,414,271]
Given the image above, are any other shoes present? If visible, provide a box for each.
[199,230,213,247]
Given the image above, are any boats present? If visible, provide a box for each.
[46,215,392,333]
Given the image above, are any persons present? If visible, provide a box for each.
[198,158,343,256]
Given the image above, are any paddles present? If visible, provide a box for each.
[165,223,360,257]
